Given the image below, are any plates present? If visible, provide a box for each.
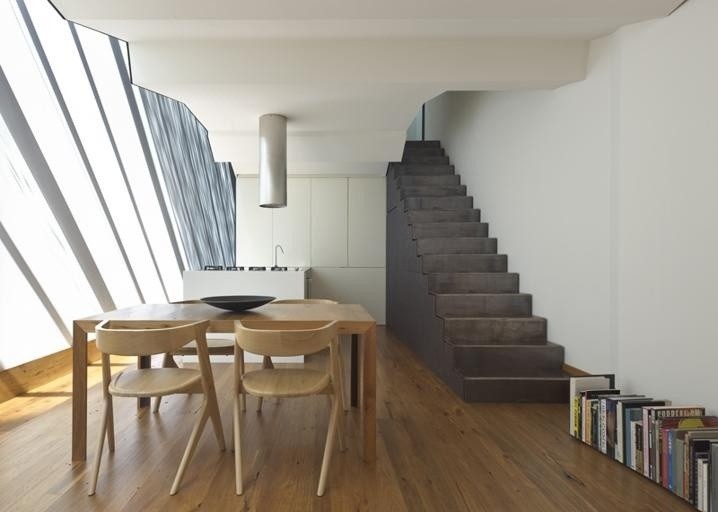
[200,295,276,312]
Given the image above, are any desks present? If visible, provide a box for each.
[72,304,377,462]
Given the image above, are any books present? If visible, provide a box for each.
[570,374,717,511]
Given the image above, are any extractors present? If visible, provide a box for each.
[258,113,287,208]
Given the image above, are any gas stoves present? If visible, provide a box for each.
[191,265,300,271]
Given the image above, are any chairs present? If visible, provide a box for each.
[232,319,346,498]
[153,300,246,414]
[256,299,348,414]
[88,318,226,496]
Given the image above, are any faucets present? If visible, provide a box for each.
[274,246,284,266]
